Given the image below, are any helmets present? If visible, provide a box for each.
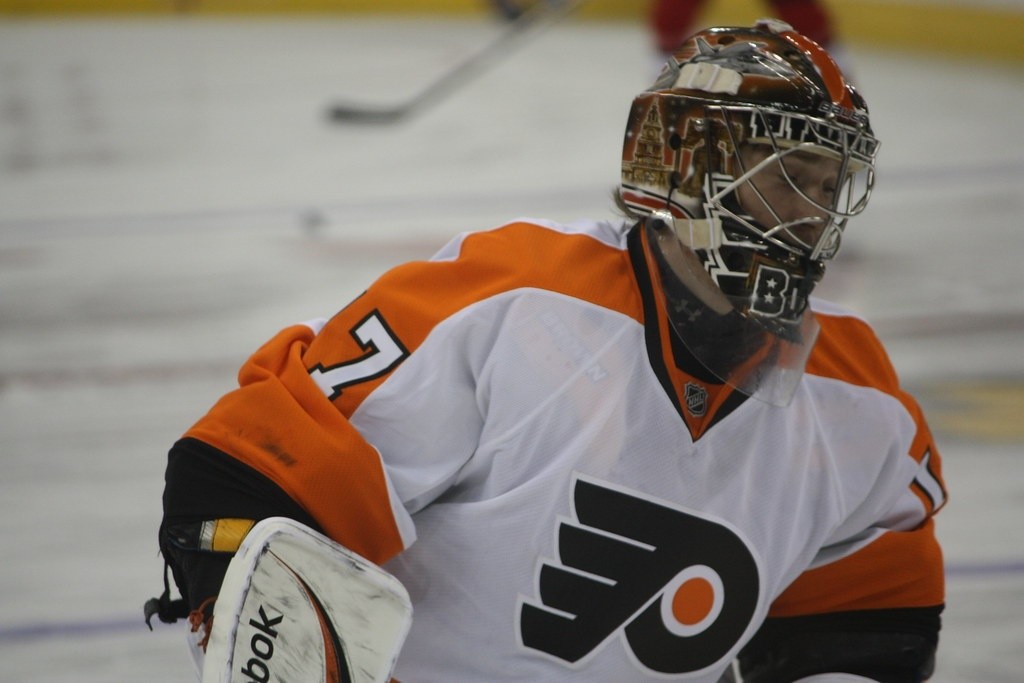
[620,17,879,222]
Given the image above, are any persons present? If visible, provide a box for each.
[145,18,947,683]
[651,0,853,91]
